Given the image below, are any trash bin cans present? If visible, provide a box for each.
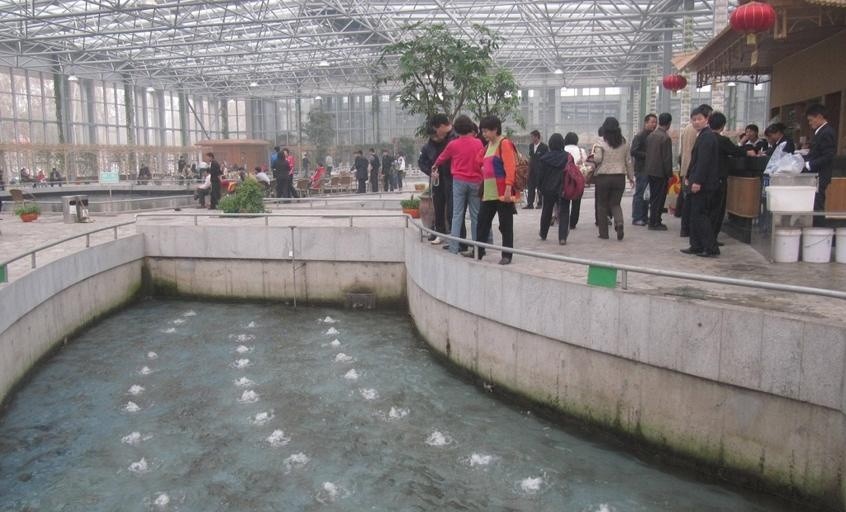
[62,195,89,223]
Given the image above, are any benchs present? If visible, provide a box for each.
[18,175,43,189]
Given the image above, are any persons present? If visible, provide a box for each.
[522,130,587,245]
[629,112,673,230]
[176,153,272,210]
[350,148,406,193]
[271,146,299,203]
[20,167,61,189]
[674,104,838,259]
[588,116,636,240]
[302,153,334,191]
[137,165,151,185]
[417,114,518,264]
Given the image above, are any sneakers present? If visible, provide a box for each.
[520,204,669,249]
[695,240,726,258]
[425,233,514,270]
[678,247,704,256]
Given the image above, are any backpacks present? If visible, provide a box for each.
[561,152,585,202]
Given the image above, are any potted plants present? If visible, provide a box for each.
[401,194,420,218]
[15,204,41,222]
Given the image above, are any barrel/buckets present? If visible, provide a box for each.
[835,227,846,264]
[586,264,617,289]
[773,227,801,263]
[802,227,834,263]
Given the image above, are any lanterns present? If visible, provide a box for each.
[729,0,776,46]
[662,71,687,96]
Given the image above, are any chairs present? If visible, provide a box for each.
[9,189,38,216]
[220,171,407,198]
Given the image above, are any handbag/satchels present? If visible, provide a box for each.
[512,160,529,193]
[575,155,597,184]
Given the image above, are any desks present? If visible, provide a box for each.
[720,175,761,226]
[644,171,680,215]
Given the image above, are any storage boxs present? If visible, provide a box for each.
[764,186,817,212]
